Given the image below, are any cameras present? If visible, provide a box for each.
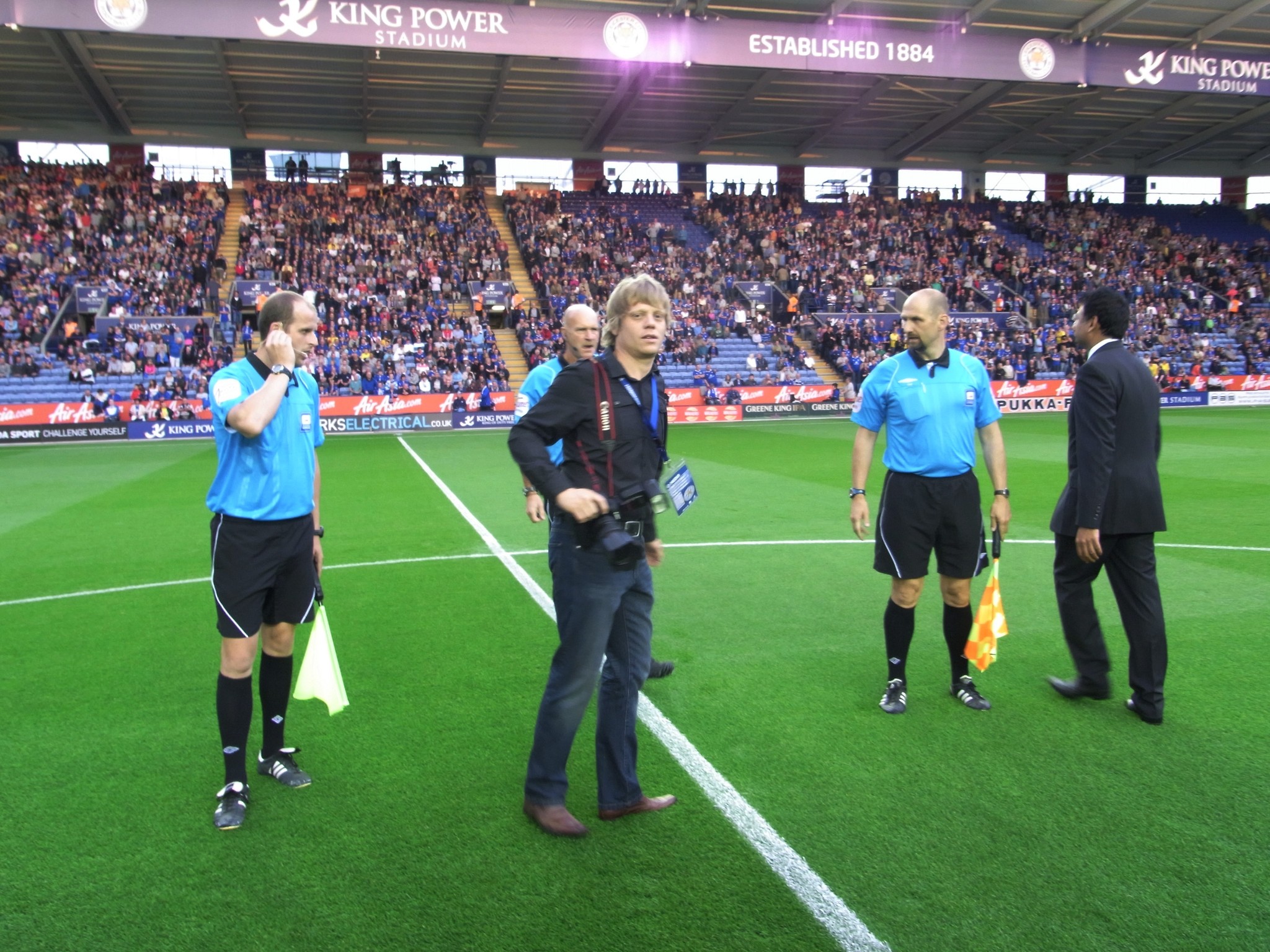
[575,496,644,571]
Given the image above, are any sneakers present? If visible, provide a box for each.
[648,659,676,679]
[881,682,908,714]
[258,745,311,789]
[950,675,992,712]
[214,781,249,829]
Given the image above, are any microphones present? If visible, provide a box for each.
[566,339,579,353]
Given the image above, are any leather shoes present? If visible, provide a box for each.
[1124,697,1165,727]
[600,791,678,819]
[1043,674,1111,704]
[524,797,590,838]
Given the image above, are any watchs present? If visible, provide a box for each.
[312,526,324,538]
[994,488,1011,499]
[521,485,537,497]
[848,487,866,499]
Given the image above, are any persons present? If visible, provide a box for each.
[0,142,1270,414]
[849,288,1014,715]
[1039,287,1177,724]
[185,288,325,831]
[510,301,675,682]
[505,274,677,846]
